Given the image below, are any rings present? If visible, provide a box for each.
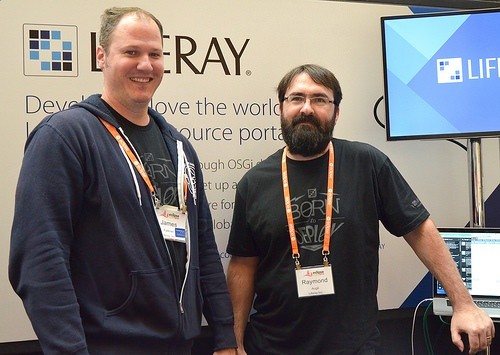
[487,336,491,339]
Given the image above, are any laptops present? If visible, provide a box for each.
[432,227,500,319]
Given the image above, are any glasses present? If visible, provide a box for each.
[280,95,337,106]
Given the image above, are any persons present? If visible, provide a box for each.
[8,7,238,355]
[226,63,495,355]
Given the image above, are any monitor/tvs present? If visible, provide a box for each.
[381,9,500,141]
